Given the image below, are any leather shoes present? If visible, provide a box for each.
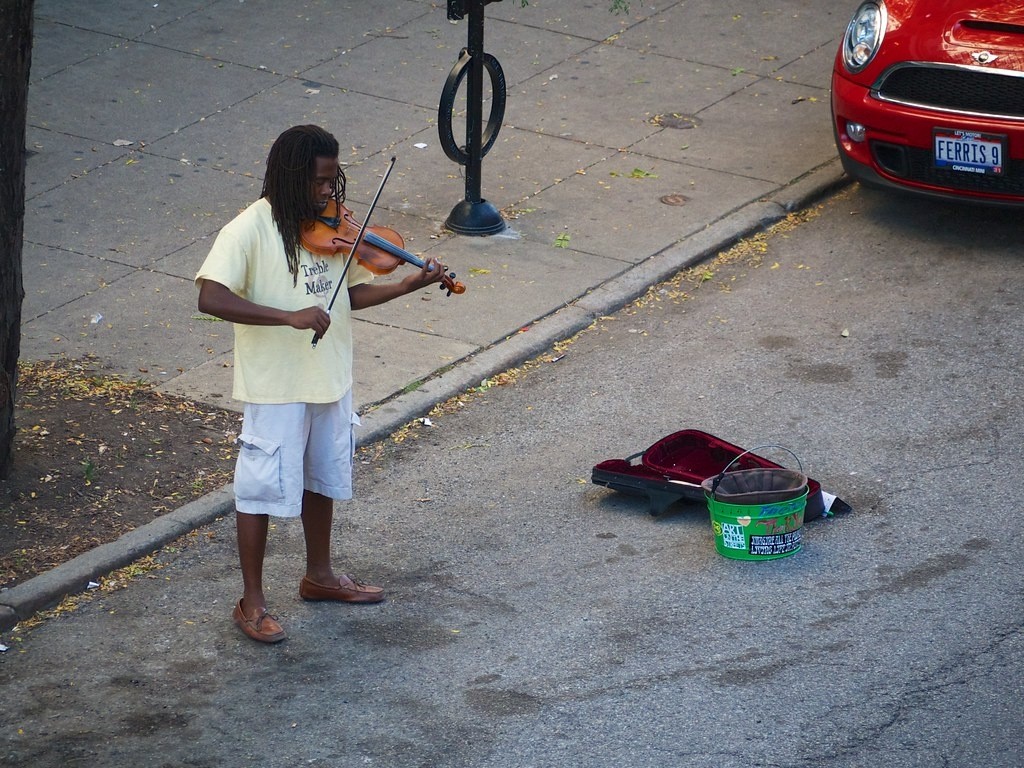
[298,575,385,603]
[232,598,286,644]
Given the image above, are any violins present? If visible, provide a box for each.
[298,198,467,298]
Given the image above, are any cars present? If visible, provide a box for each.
[827,0,1024,209]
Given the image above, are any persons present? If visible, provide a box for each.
[193,124,447,644]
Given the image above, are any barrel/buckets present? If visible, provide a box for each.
[703,445,809,561]
